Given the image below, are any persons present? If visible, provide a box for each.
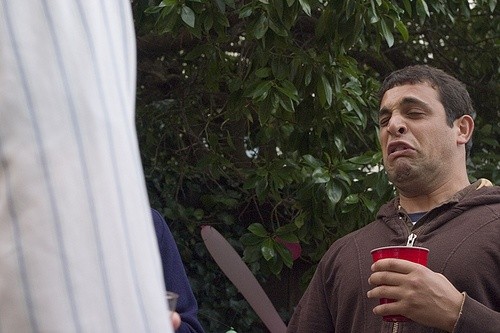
[287,62,500,333]
[0,0,205,333]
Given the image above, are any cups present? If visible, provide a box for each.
[167,292,179,321]
[370,246,430,322]
